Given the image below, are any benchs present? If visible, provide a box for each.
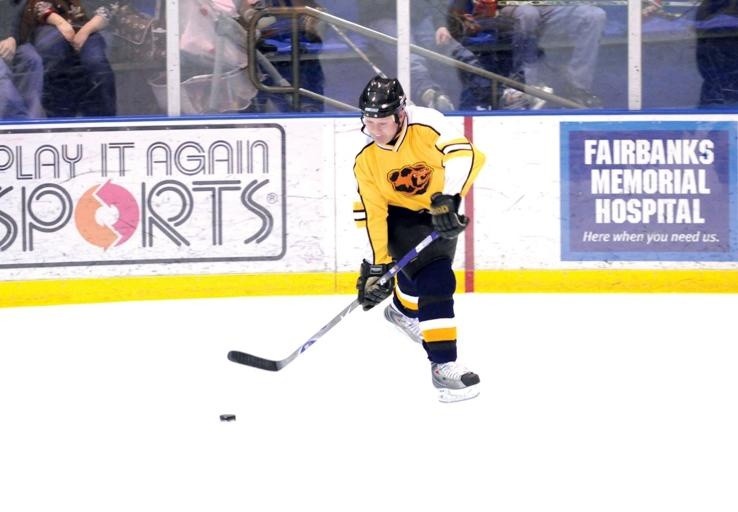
[89,1,736,119]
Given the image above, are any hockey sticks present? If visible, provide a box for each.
[228,228,439,370]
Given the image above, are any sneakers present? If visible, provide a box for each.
[431,360,480,390]
[423,90,454,112]
[500,87,550,112]
[383,303,424,344]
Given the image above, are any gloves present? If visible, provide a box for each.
[431,192,469,241]
[356,259,397,311]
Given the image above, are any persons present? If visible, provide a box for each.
[0,0,118,119]
[358,0,608,109]
[352,77,488,403]
[231,1,324,113]
[695,0,738,107]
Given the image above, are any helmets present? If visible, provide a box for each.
[358,75,406,118]
